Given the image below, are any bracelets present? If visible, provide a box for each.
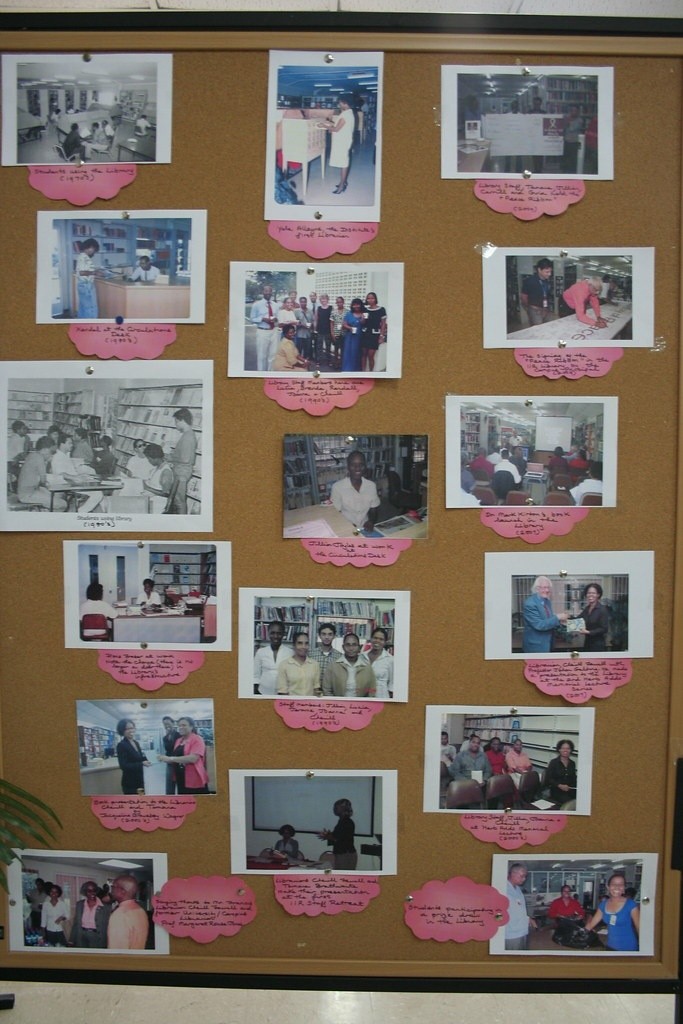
[379,334,384,337]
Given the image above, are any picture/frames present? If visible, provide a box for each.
[0,11,683,994]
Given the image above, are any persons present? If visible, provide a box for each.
[250,286,388,372]
[440,731,578,802]
[25,872,150,949]
[8,407,199,514]
[460,430,603,505]
[116,716,209,795]
[521,258,619,329]
[50,105,156,161]
[464,96,585,174]
[254,620,394,700]
[330,450,381,533]
[316,93,369,195]
[128,255,161,282]
[77,578,162,641]
[274,798,359,871]
[75,237,106,318]
[505,862,641,952]
[522,575,609,653]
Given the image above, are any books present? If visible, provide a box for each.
[283,440,312,490]
[254,601,400,654]
